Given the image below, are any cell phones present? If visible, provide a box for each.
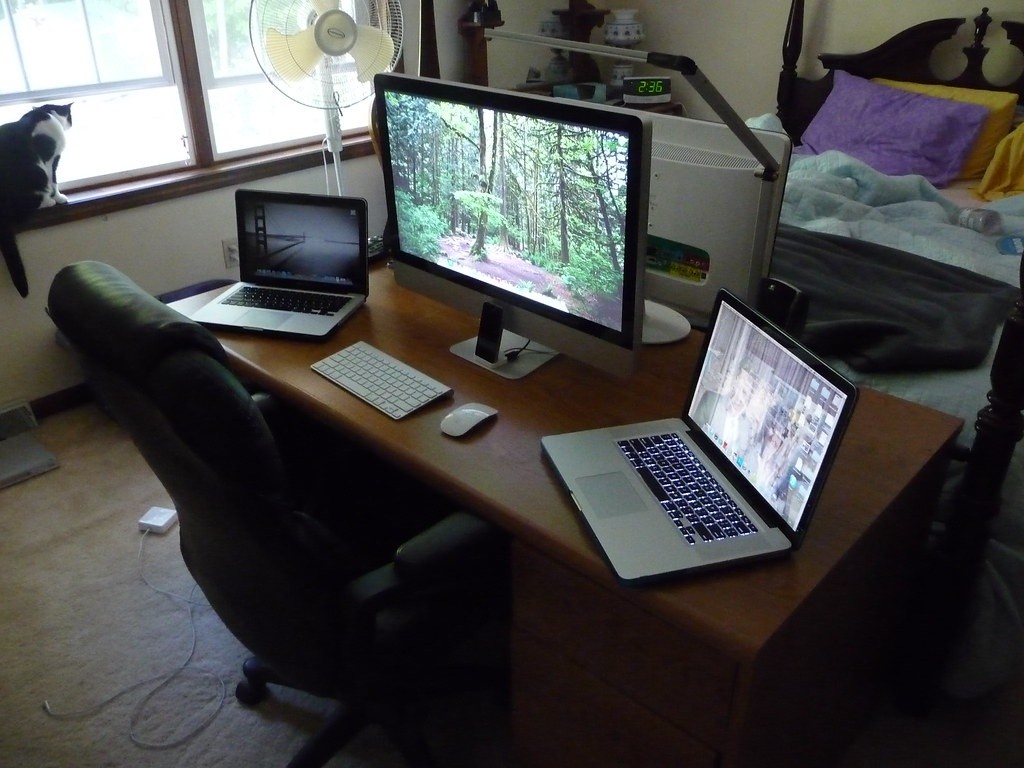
[475,302,506,364]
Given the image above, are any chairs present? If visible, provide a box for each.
[42,260,506,765]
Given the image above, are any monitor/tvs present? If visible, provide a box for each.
[370,71,654,382]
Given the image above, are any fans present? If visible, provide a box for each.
[247,1,406,253]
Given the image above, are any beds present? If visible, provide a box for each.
[735,1,1024,691]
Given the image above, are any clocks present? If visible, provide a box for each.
[621,76,673,104]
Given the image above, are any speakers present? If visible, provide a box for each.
[751,278,809,343]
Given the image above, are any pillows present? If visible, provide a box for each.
[800,65,1019,196]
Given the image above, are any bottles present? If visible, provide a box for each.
[949,207,1001,236]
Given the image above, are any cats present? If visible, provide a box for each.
[0,99,75,298]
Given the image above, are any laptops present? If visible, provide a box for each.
[539,287,861,584]
[189,188,370,343]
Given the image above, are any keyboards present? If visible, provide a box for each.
[310,341,454,422]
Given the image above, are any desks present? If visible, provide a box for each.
[158,239,969,768]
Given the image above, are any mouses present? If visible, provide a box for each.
[440,401,499,437]
